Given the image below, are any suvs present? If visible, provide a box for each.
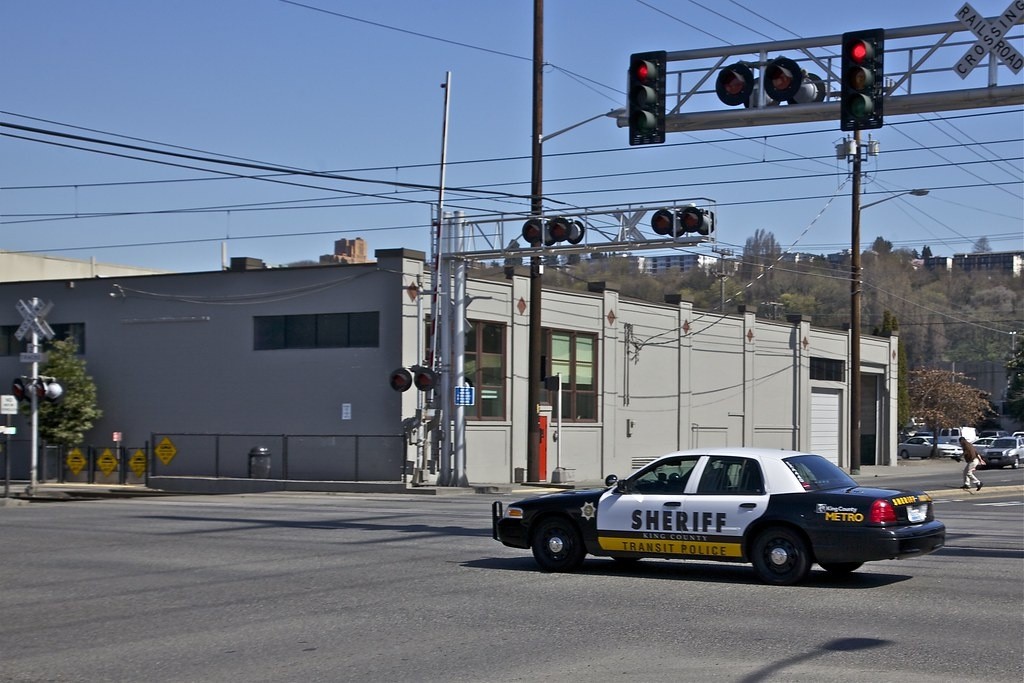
[982,436,1024,470]
[979,430,1011,439]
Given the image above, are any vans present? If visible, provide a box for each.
[1012,431,1024,437]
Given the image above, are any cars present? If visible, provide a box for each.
[492,447,947,586]
[913,432,935,436]
[972,437,997,457]
[897,436,964,460]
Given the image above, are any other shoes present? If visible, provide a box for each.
[976,482,983,491]
[960,485,971,489]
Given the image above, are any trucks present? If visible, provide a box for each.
[939,426,977,444]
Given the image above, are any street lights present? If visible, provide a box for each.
[527,106,628,482]
[850,189,931,475]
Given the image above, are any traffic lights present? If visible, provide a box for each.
[548,217,571,242]
[12,377,26,401]
[36,377,46,401]
[389,368,412,392]
[680,206,704,233]
[763,57,802,102]
[651,210,674,236]
[414,367,437,391]
[716,63,754,107]
[841,28,885,132]
[629,50,668,146]
[522,219,542,243]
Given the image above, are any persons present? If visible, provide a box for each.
[958,437,986,491]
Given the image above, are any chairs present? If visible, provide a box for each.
[677,464,731,492]
